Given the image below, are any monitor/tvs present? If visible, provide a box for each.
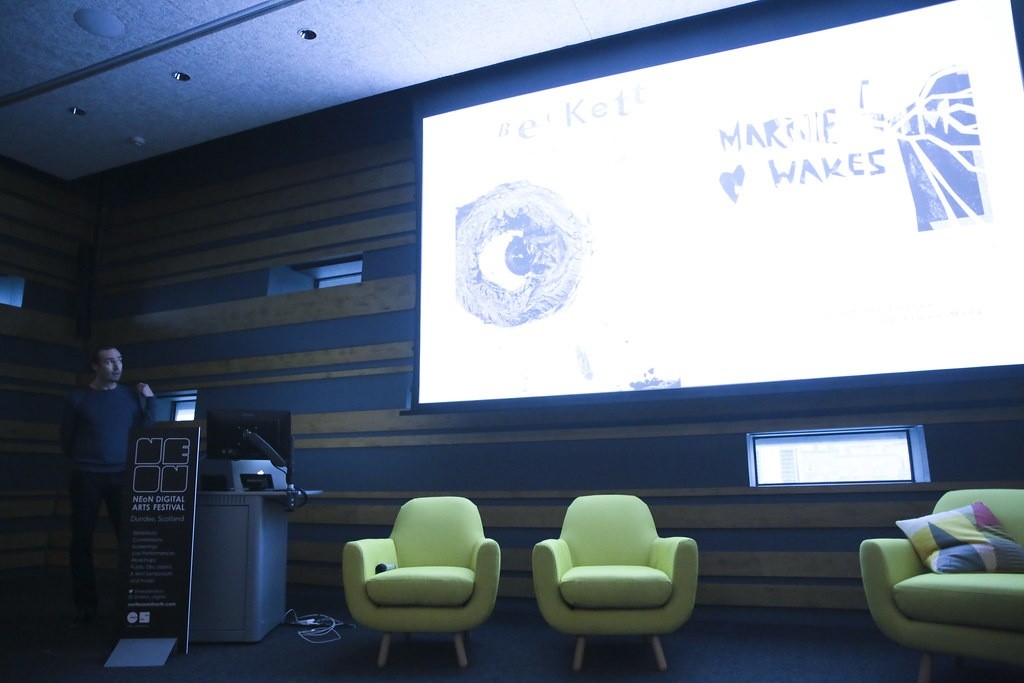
[208,409,292,461]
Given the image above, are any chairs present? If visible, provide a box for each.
[342,495,501,669]
[533,493,700,671]
[860,489,1024,683]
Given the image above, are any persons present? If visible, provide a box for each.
[55,341,157,629]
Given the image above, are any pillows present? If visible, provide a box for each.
[895,501,1024,575]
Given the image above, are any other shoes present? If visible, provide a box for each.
[72,611,104,625]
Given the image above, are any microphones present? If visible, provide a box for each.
[375,563,396,574]
